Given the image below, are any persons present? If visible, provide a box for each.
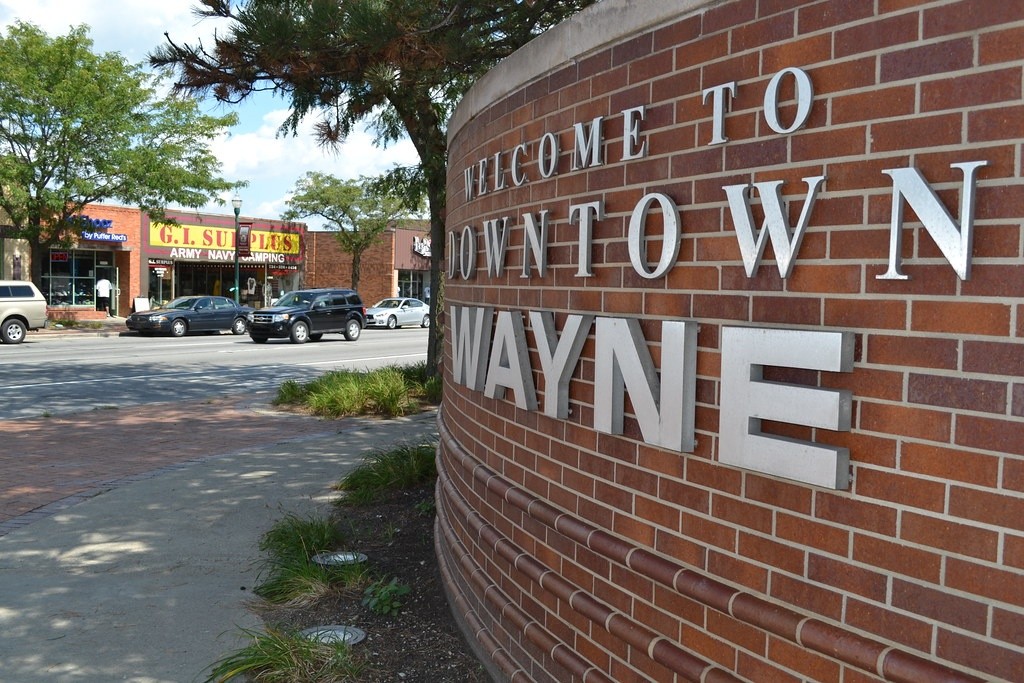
[424,285,430,305]
[96,274,112,317]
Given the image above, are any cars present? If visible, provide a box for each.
[125,295,256,337]
[0,281,49,344]
[367,297,430,329]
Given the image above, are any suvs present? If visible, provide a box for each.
[246,285,367,344]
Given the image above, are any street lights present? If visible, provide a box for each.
[232,187,243,304]
